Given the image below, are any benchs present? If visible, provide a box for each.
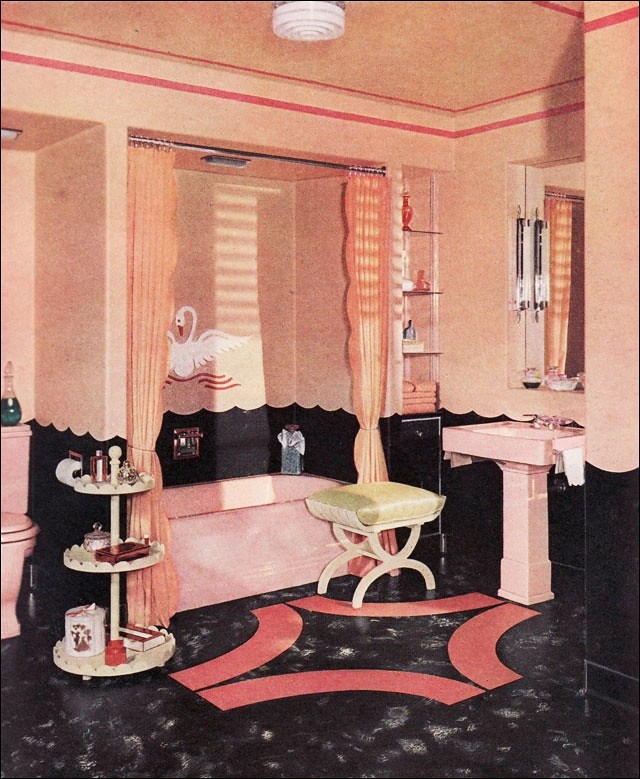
[162,471,352,612]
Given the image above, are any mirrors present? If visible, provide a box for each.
[505,147,584,392]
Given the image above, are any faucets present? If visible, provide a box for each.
[523,414,562,431]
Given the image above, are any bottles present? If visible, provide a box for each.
[403,320,416,340]
[118,460,136,485]
[402,195,413,231]
[414,270,431,292]
[0,361,21,427]
[89,450,108,483]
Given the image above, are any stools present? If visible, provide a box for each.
[305,481,447,608]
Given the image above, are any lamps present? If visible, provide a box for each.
[272,1,347,42]
[514,205,528,316]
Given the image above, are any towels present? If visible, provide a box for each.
[554,448,586,486]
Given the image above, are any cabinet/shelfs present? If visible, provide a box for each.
[53,445,178,681]
[401,231,443,537]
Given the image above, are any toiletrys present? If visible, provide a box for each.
[544,366,562,385]
[521,368,541,388]
[280,424,304,475]
[403,319,417,341]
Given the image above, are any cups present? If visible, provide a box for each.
[83,522,111,552]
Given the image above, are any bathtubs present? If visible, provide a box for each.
[158,471,350,612]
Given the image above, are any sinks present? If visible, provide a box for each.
[441,420,586,467]
